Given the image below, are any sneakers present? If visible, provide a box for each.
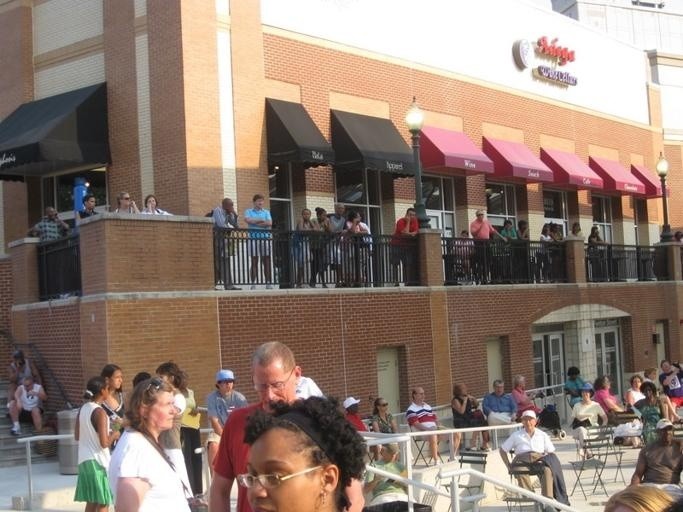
[343,397,360,411]
[475,279,558,285]
[215,282,371,290]
[579,450,595,459]
[11,425,20,433]
[433,446,495,467]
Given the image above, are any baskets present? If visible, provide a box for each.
[32,429,57,457]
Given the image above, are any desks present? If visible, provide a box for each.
[437,248,550,285]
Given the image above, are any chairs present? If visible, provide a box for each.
[366,422,380,466]
[408,424,444,468]
[440,452,488,511]
[587,424,626,486]
[568,437,610,501]
[504,449,539,510]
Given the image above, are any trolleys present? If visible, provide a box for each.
[537,387,566,440]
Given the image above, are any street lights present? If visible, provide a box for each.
[655,151,675,242]
[404,96,432,229]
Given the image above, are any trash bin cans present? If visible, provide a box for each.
[57,408,81,475]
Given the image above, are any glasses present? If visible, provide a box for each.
[377,403,388,407]
[236,464,327,490]
[143,376,163,401]
[250,365,296,391]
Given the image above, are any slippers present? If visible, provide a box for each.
[673,417,683,425]
[631,443,644,449]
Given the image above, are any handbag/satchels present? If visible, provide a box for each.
[472,409,484,419]
[571,418,592,430]
[185,492,210,512]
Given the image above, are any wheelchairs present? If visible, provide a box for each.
[446,241,479,284]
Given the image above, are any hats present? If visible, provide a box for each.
[12,350,24,357]
[519,409,536,420]
[579,383,595,398]
[383,443,401,453]
[215,370,234,384]
[656,419,672,430]
